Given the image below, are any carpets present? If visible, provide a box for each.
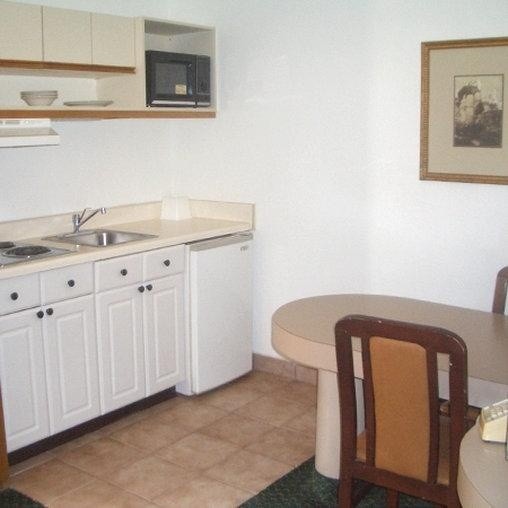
[0,487,46,506]
[238,456,455,506]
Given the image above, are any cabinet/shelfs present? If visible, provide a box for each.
[2,1,219,120]
[2,259,102,454]
[95,240,186,419]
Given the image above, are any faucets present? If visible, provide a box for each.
[80,207,108,225]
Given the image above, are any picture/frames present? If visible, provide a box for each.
[417,37,507,187]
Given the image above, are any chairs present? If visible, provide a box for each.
[332,312,486,507]
[491,266,508,315]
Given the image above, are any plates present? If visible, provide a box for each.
[62,98,115,109]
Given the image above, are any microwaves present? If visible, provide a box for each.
[146,50,211,108]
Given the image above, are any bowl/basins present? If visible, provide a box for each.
[19,89,58,107]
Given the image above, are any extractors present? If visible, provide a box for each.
[1,118,61,149]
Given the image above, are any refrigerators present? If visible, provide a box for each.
[173,231,251,396]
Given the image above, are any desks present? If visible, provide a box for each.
[456,418,508,508]
[269,291,508,481]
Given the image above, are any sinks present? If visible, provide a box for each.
[41,228,161,247]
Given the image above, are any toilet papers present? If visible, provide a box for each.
[160,190,191,222]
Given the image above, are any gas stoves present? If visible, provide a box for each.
[0,238,76,270]
